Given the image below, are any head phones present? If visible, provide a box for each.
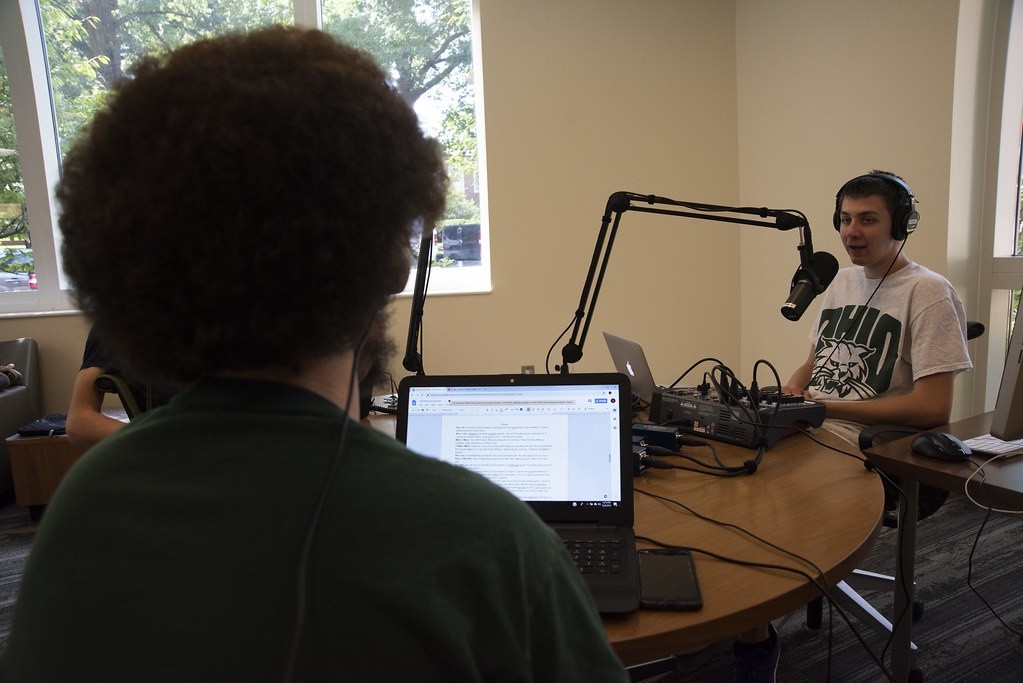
[832,173,921,241]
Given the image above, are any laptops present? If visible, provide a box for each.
[396,372,640,620]
[602,331,657,404]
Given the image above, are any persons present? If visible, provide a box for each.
[788,169,974,427]
[7,20,630,683]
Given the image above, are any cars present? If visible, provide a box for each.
[0,248,38,295]
[411,219,481,260]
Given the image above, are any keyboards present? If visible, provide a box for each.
[962,433,1023,459]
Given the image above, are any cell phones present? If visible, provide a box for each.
[636,547,703,612]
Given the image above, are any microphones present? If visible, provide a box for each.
[780,251,839,321]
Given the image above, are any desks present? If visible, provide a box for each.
[356,389,884,683]
[862,409,1023,683]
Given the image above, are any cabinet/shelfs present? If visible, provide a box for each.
[5,427,93,523]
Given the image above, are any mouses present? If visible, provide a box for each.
[911,430,973,463]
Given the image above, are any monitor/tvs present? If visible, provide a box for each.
[989,288,1023,441]
